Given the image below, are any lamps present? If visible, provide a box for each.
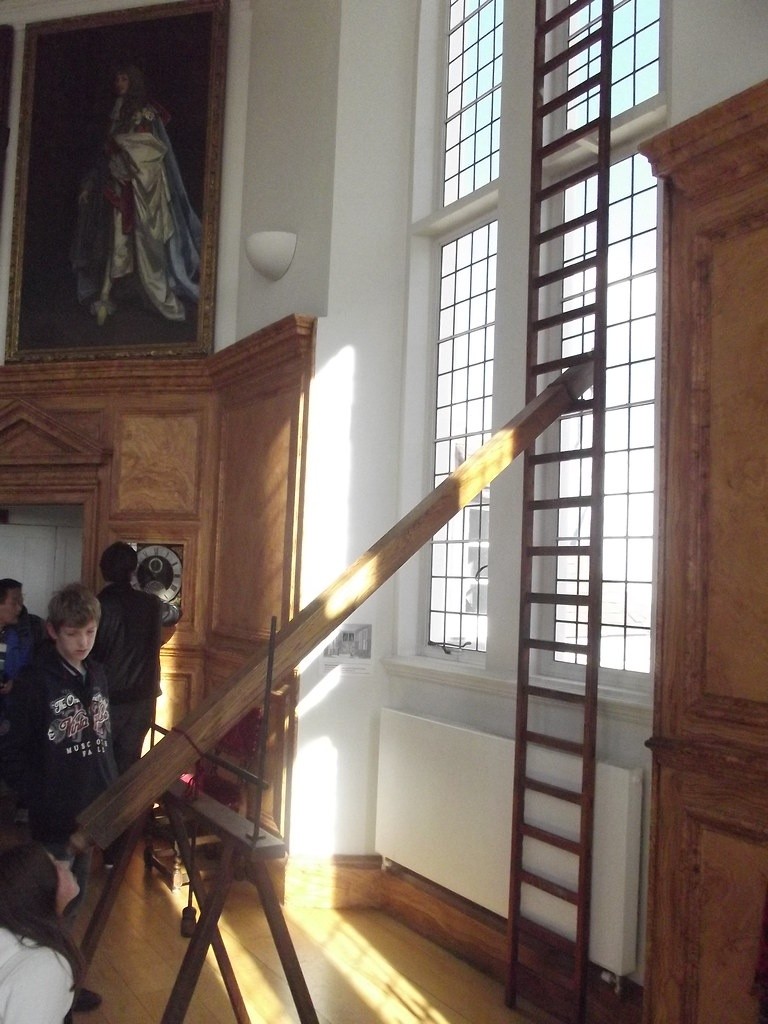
[244,231,298,283]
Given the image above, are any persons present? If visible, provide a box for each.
[0,843,82,1024]
[0,578,135,1012]
[89,542,183,867]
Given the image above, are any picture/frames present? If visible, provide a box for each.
[3,0,231,371]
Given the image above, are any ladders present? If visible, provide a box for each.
[505,1,618,1024]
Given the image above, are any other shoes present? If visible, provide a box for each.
[71,989,102,1012]
[103,854,113,870]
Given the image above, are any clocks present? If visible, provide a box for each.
[128,544,185,605]
